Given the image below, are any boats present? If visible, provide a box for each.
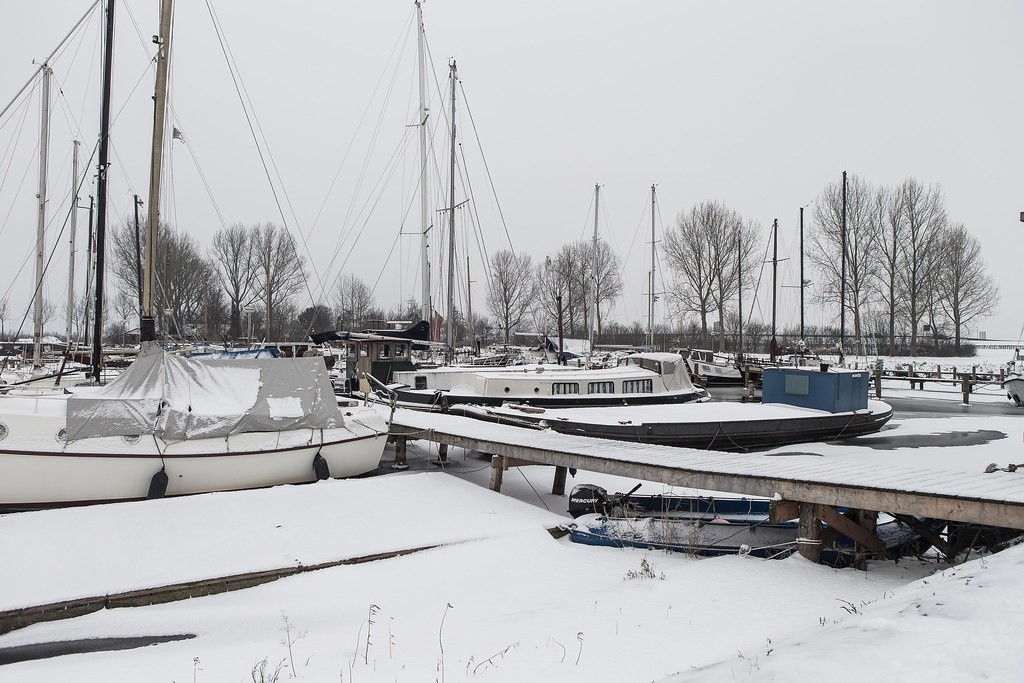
[568,512,948,571]
[450,365,895,456]
[1002,350,1024,400]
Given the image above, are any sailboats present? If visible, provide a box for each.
[0,2,390,507]
[326,325,712,403]
